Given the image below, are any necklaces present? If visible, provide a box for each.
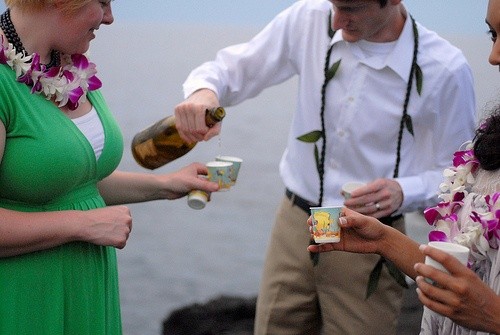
[1,13,103,111]
[423,119,500,260]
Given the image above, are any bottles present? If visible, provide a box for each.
[130,106,226,170]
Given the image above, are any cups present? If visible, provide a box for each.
[186,174,210,209]
[342,181,367,200]
[204,161,233,191]
[310,206,342,243]
[425,240,470,289]
[215,155,243,185]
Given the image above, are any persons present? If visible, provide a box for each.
[174,0,476,335]
[0,0,219,335]
[309,0,500,335]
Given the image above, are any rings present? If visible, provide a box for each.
[374,202,382,211]
